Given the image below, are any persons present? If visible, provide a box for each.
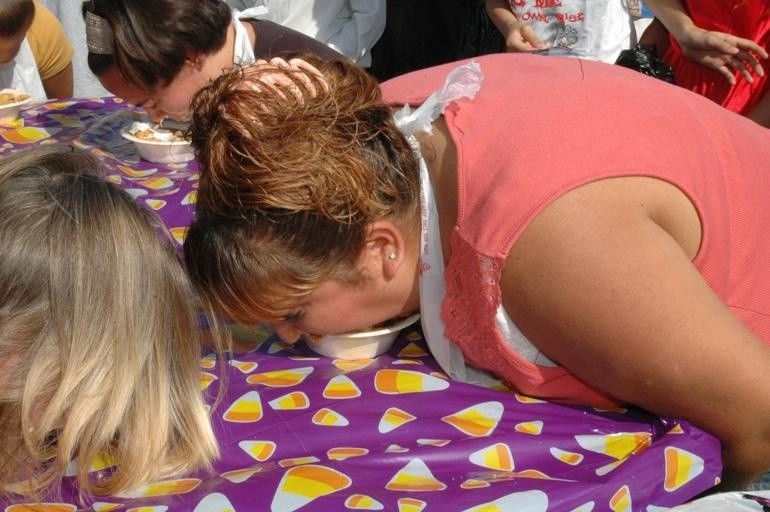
[0,142,235,505]
[181,49,770,499]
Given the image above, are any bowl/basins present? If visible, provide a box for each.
[119,120,197,164]
[300,314,422,360]
[0,88,33,127]
